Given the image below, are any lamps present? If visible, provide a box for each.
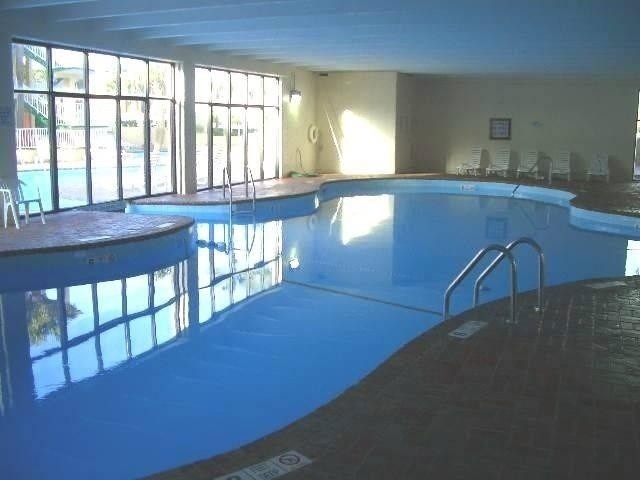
[290,90,301,104]
[288,258,300,269]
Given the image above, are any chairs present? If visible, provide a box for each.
[485,149,511,177]
[455,148,481,176]
[516,149,538,180]
[551,150,571,182]
[587,152,610,184]
[0,177,47,230]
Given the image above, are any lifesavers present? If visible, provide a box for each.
[308,124,319,143]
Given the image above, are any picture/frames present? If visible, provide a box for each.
[489,117,511,140]
